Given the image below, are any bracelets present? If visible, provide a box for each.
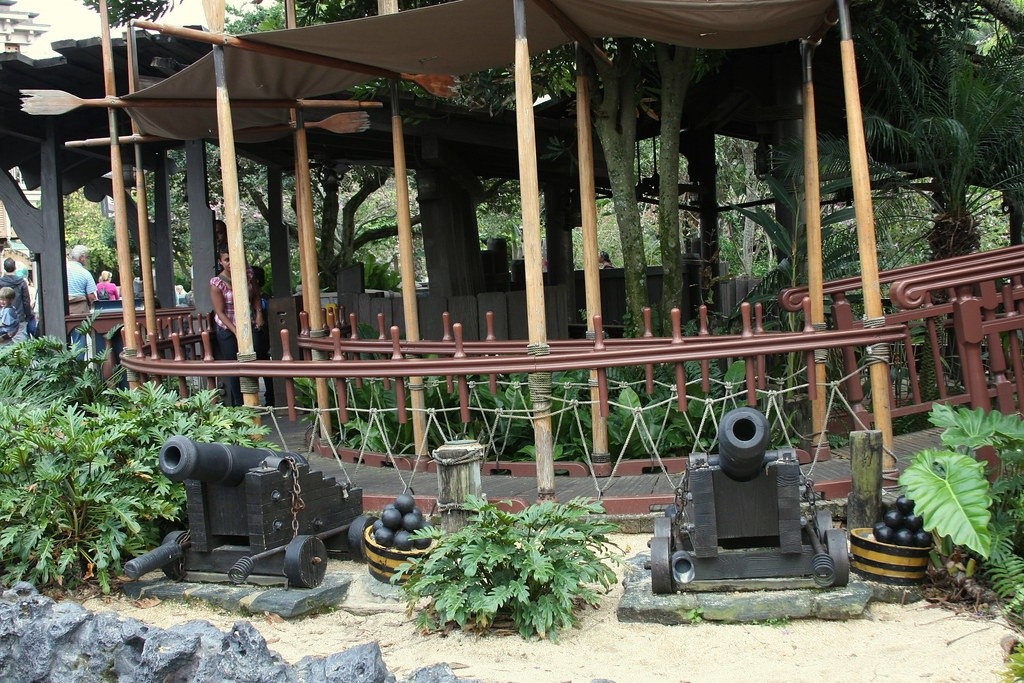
[256,308,262,312]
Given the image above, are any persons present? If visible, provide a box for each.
[0,287,19,343]
[66,244,106,369]
[0,257,33,344]
[599,251,613,269]
[210,246,264,409]
[96,270,120,301]
[249,264,274,407]
[213,219,227,278]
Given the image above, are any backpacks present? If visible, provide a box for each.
[98,283,109,300]
